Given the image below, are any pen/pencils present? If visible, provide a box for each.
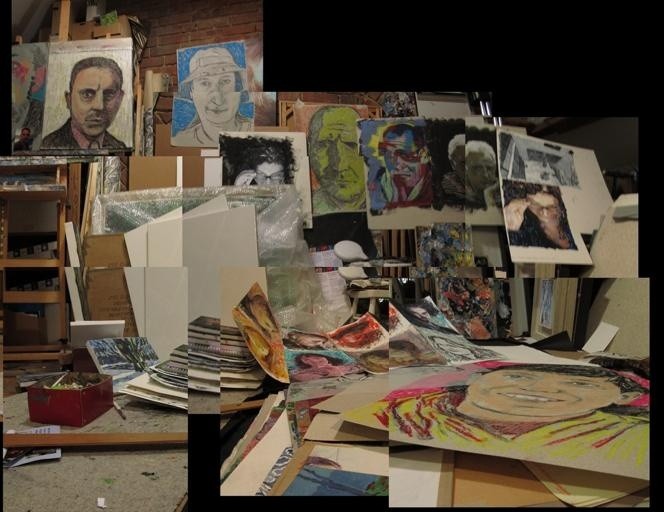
[113,402,127,420]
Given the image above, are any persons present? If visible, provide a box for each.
[442,134,466,201]
[41,56,127,150]
[233,144,290,186]
[503,177,578,250]
[465,141,500,215]
[14,128,33,152]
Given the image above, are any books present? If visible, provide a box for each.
[69,320,125,347]
[220,325,265,389]
[117,343,188,412]
[86,337,161,396]
[188,312,220,395]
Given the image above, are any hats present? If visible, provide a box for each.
[179,47,246,98]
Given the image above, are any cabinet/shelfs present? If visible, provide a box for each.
[1,163,67,360]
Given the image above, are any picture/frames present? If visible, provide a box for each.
[528,278,580,342]
